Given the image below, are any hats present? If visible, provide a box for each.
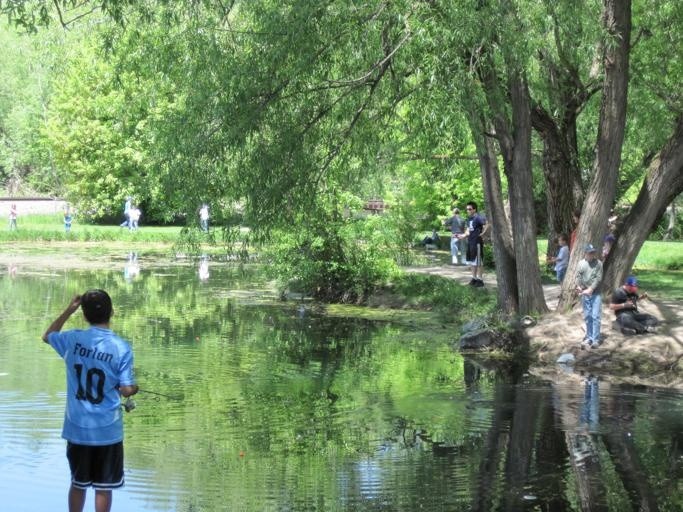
[584,244,596,253]
[625,277,637,292]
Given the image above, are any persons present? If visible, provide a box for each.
[198,258,209,285]
[443,207,466,265]
[129,253,141,280]
[573,243,605,347]
[463,358,480,409]
[546,233,569,298]
[125,251,132,286]
[568,210,581,257]
[63,210,72,228]
[119,196,131,227]
[611,381,658,423]
[198,203,210,233]
[8,204,17,230]
[573,377,602,471]
[609,275,660,335]
[453,201,489,288]
[127,204,141,232]
[41,288,139,511]
[602,225,616,263]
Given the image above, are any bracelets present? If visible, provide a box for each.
[622,303,624,308]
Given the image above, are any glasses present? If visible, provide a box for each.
[467,208,472,210]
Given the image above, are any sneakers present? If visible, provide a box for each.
[582,338,602,349]
[621,327,637,334]
[646,325,657,333]
[470,278,483,287]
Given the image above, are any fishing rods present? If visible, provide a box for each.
[552,289,581,293]
[137,388,183,402]
